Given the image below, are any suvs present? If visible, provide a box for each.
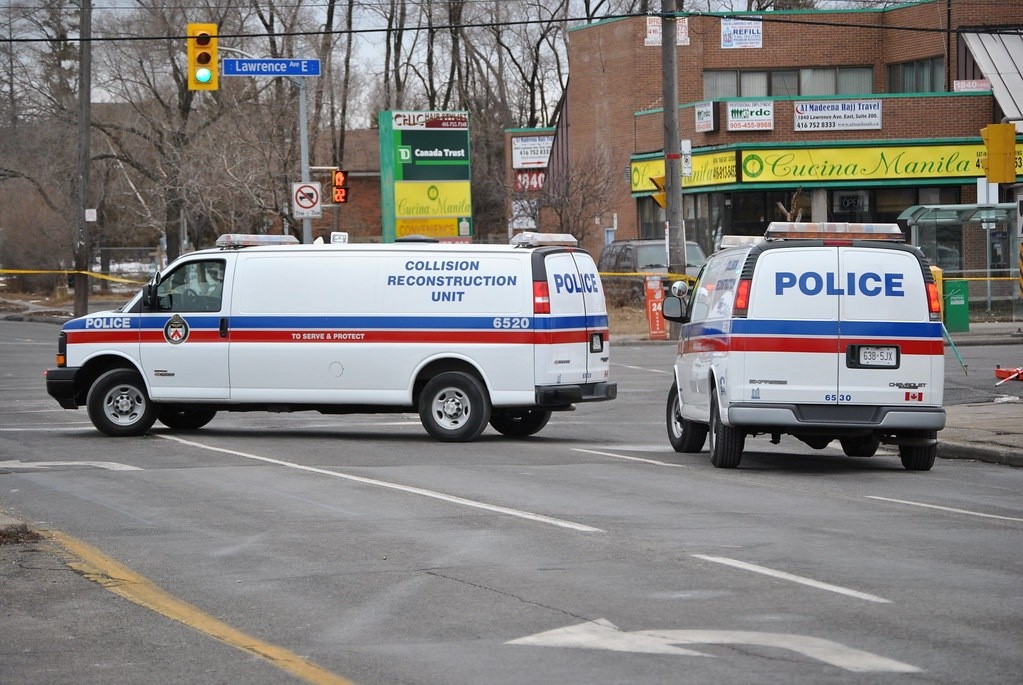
[43,231,618,441]
[660,216,945,466]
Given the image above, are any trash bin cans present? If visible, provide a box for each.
[943,280,969,332]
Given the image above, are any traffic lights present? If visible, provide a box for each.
[331,170,347,204]
[185,20,220,96]
